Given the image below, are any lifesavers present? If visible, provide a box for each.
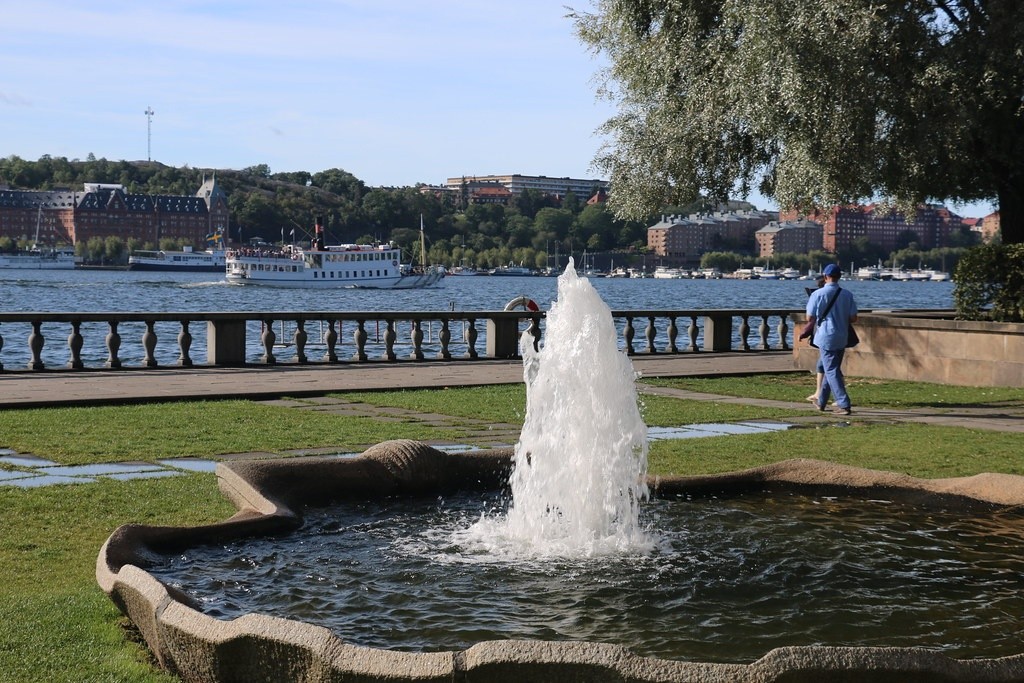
[293,254,298,260]
[504,297,540,335]
[393,261,397,266]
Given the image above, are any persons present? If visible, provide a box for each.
[232,246,290,260]
[799,264,858,415]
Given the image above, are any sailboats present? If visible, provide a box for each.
[450,234,479,276]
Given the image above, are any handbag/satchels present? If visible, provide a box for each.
[845,323,861,348]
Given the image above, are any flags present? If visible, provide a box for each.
[205,230,223,245]
[587,194,597,204]
[290,229,294,235]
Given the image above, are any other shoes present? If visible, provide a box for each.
[832,408,851,414]
[813,400,824,412]
[806,395,819,401]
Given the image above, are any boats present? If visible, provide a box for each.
[858,258,952,281]
[533,240,823,280]
[489,260,530,276]
[127,234,233,272]
[225,212,448,289]
[0,204,77,271]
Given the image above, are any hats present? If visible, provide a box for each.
[823,264,841,276]
